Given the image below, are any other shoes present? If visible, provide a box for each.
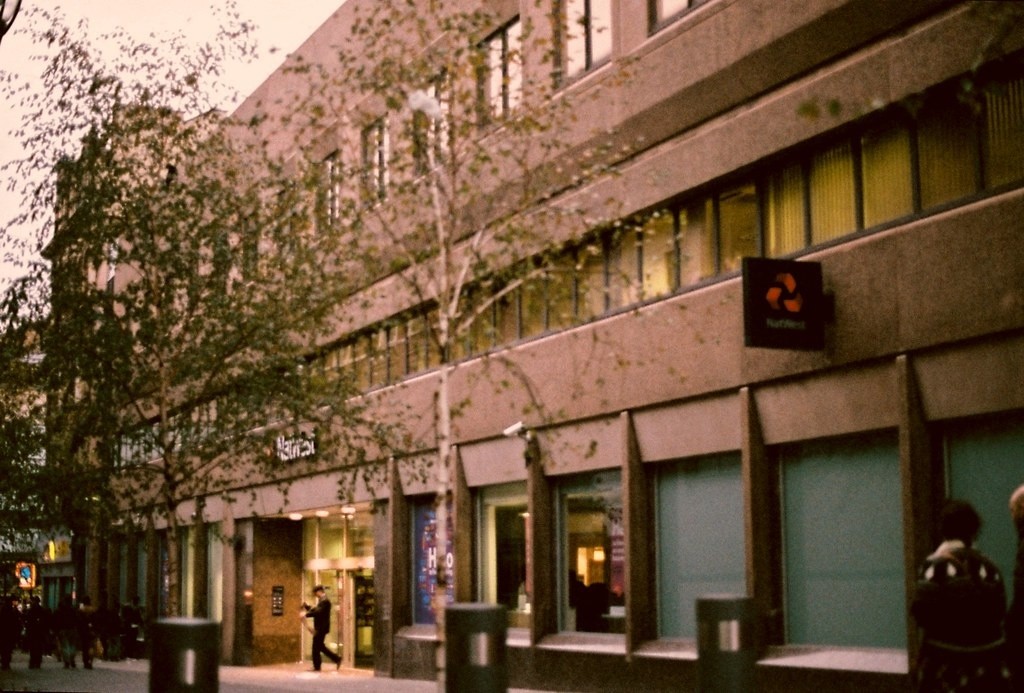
[336,657,342,670]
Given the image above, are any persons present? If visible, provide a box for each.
[518,574,525,610]
[905,501,1006,693]
[27,596,50,669]
[123,596,143,657]
[303,585,342,672]
[51,594,121,670]
[0,600,25,670]
[1008,483,1024,693]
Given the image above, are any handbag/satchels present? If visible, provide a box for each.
[55,618,76,637]
[94,636,104,657]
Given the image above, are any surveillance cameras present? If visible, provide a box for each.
[504,421,524,437]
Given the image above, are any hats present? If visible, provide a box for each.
[30,596,41,602]
[312,585,322,593]
